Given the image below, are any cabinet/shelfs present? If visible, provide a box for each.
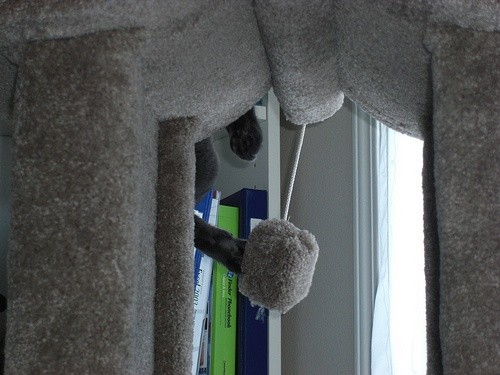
[190,87,282,375]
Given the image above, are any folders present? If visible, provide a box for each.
[193,187,270,374]
